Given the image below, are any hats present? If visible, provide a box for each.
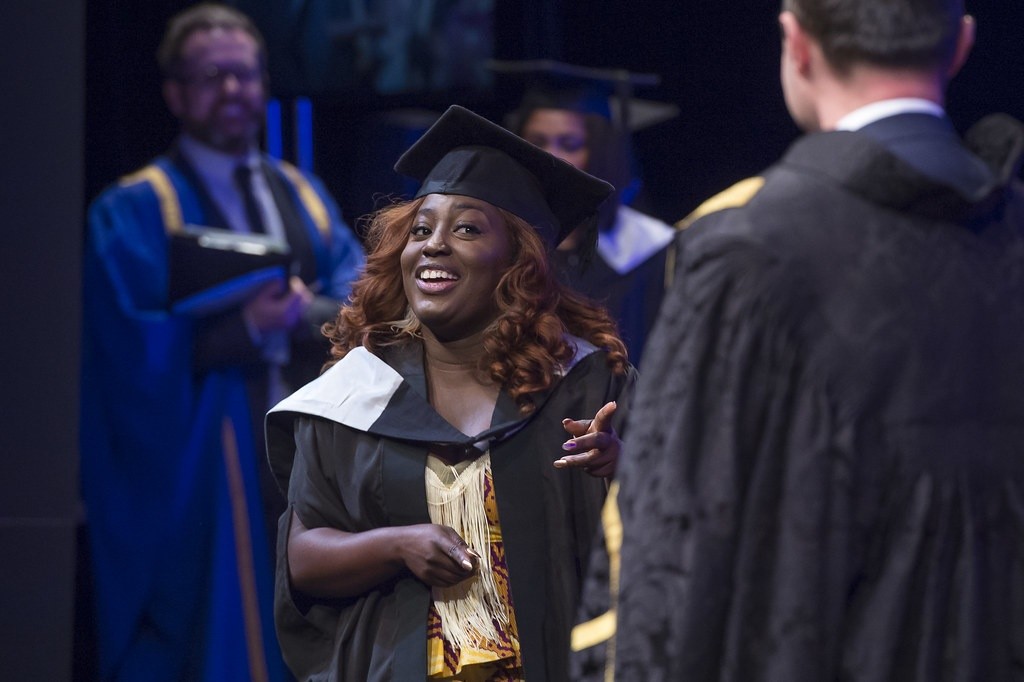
[476,57,662,125]
[392,104,616,254]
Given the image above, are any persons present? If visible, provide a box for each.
[571,1,1024,682]
[486,59,676,377]
[80,5,364,682]
[264,104,639,682]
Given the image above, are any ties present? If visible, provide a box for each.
[233,161,269,234]
